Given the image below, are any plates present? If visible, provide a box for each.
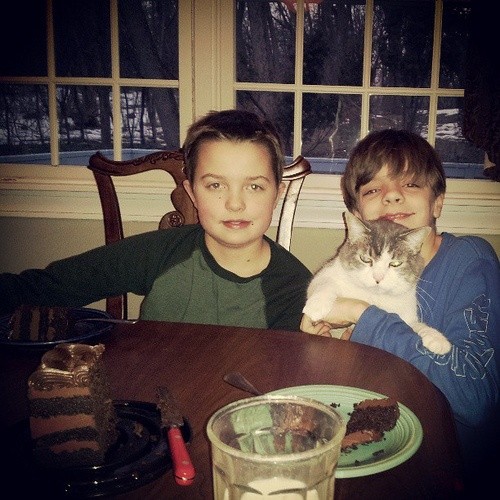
[227,383,422,480]
[2,401,191,500]
[0,308,115,352]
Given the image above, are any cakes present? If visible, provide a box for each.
[267,396,400,451]
[26,341,118,463]
[6,303,69,341]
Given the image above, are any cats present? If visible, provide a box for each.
[307,211,451,355]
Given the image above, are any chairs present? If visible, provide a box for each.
[86,150,312,319]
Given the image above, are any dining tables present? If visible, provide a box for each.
[0,319,457,500]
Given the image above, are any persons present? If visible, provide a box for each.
[300,129,500,428]
[0,108,314,332]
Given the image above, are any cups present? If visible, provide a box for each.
[206,394,347,500]
